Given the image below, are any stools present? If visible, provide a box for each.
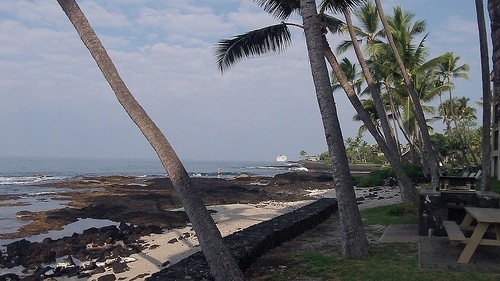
[442,218,466,244]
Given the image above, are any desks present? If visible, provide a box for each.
[448,205,500,264]
